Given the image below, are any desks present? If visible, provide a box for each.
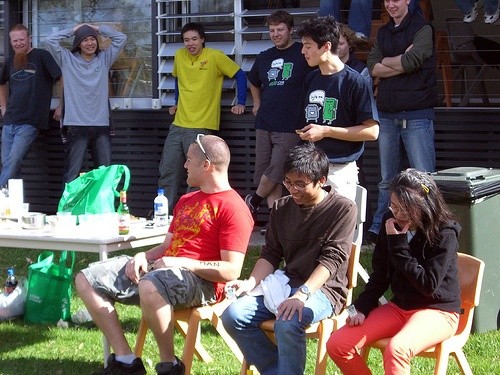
[0,213,174,369]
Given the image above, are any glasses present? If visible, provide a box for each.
[194,134,207,158]
[388,201,416,215]
[282,176,316,190]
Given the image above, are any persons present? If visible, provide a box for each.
[154,22,247,214]
[295,16,379,202]
[43,23,128,215]
[326,168,462,375]
[316,0,373,50]
[454,0,500,24]
[335,22,379,99]
[75,134,255,375]
[0,24,64,190]
[244,8,321,234]
[363,0,438,245]
[222,143,358,375]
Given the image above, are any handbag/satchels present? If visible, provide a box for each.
[24,250,76,324]
[57,164,130,215]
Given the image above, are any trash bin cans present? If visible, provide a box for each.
[428,167,500,334]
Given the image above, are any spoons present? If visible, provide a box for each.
[145,217,170,226]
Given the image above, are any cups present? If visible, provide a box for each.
[152,99,161,109]
[123,99,132,109]
[56,212,71,229]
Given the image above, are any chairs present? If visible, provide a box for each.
[351,185,387,309]
[435,31,492,107]
[87,22,150,98]
[240,241,360,375]
[133,278,261,375]
[359,252,485,375]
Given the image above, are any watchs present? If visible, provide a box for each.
[300,284,311,301]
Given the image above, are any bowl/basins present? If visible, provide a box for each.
[19,212,45,228]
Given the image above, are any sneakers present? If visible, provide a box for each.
[92,354,146,375]
[244,194,259,224]
[156,355,185,375]
[484,0,500,23]
[463,0,485,23]
[354,32,369,43]
[260,224,268,234]
[363,230,379,246]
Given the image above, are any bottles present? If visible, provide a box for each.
[154,189,168,232]
[117,190,129,235]
[5,269,18,294]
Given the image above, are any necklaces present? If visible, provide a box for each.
[187,49,203,66]
